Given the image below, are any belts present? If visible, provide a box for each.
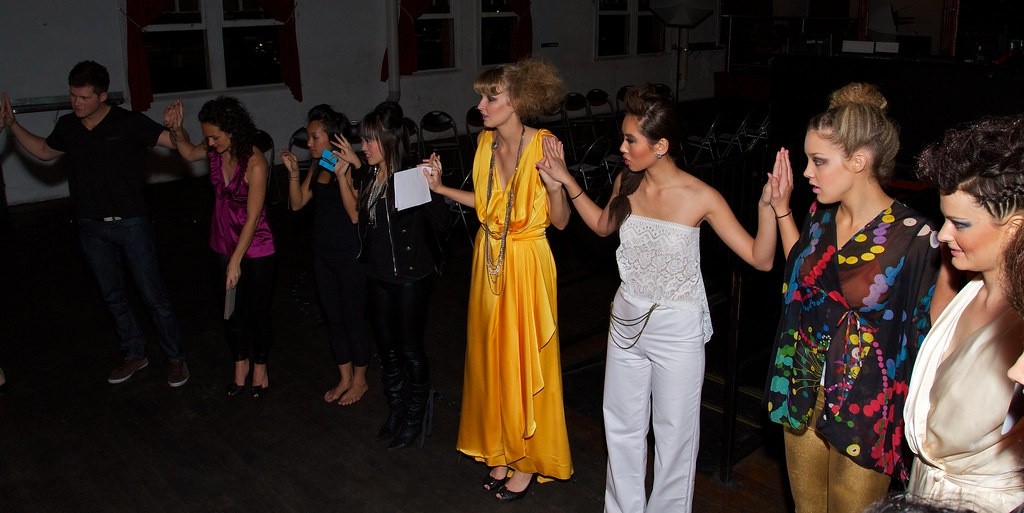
[97,215,129,222]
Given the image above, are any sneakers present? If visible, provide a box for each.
[107,355,149,384]
[167,359,190,387]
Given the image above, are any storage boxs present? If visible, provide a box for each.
[842,40,899,54]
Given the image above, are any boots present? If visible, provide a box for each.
[387,351,434,451]
[372,353,407,443]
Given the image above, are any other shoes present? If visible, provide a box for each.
[245,373,265,408]
[223,367,252,401]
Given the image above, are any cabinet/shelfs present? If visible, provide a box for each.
[769,53,1024,152]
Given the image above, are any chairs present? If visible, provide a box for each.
[250,84,774,251]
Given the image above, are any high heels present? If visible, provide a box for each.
[481,465,510,491]
[495,474,537,502]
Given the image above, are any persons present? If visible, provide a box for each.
[532,83,789,513]
[903,115,1024,513]
[770,82,947,513]
[0,61,441,452]
[415,59,575,502]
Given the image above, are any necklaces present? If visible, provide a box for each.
[486,124,528,295]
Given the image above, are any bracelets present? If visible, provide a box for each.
[290,173,300,178]
[777,211,791,219]
[289,179,300,182]
[571,188,584,199]
